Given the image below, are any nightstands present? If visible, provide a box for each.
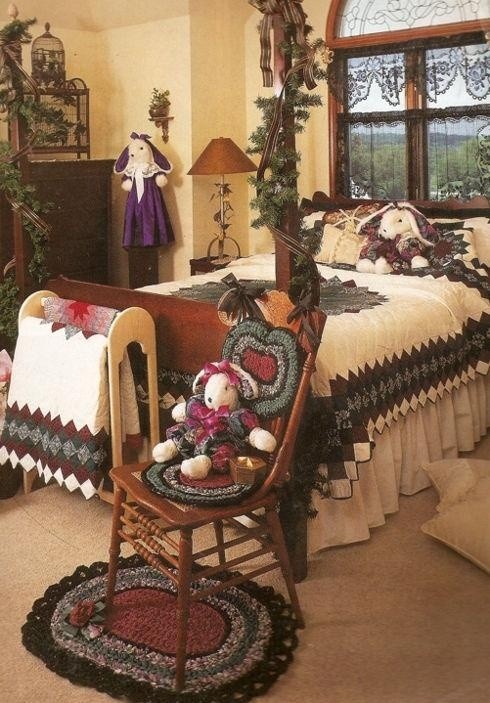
[191,254,232,279]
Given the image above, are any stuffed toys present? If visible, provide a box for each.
[152,359,279,480]
[112,130,176,252]
[354,199,439,274]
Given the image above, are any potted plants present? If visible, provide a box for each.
[0,274,29,501]
[146,88,171,118]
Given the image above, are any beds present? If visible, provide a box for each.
[44,192,490,558]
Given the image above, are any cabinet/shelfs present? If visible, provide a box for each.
[0,159,115,352]
[5,77,91,160]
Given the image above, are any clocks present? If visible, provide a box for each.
[187,136,256,257]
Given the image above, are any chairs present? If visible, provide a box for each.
[104,291,326,692]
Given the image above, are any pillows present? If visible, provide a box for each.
[309,224,364,268]
[419,456,490,510]
[420,500,490,575]
[440,228,481,270]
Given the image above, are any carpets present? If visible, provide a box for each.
[22,552,297,702]
